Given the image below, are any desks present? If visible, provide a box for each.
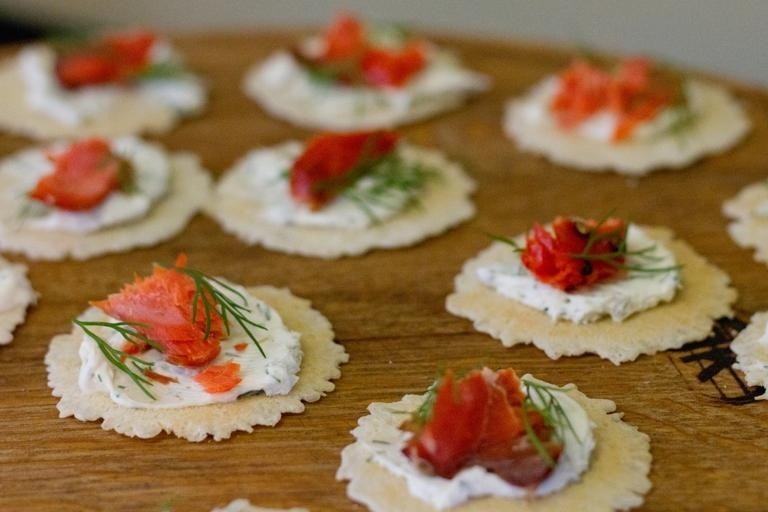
[3,29,767,510]
